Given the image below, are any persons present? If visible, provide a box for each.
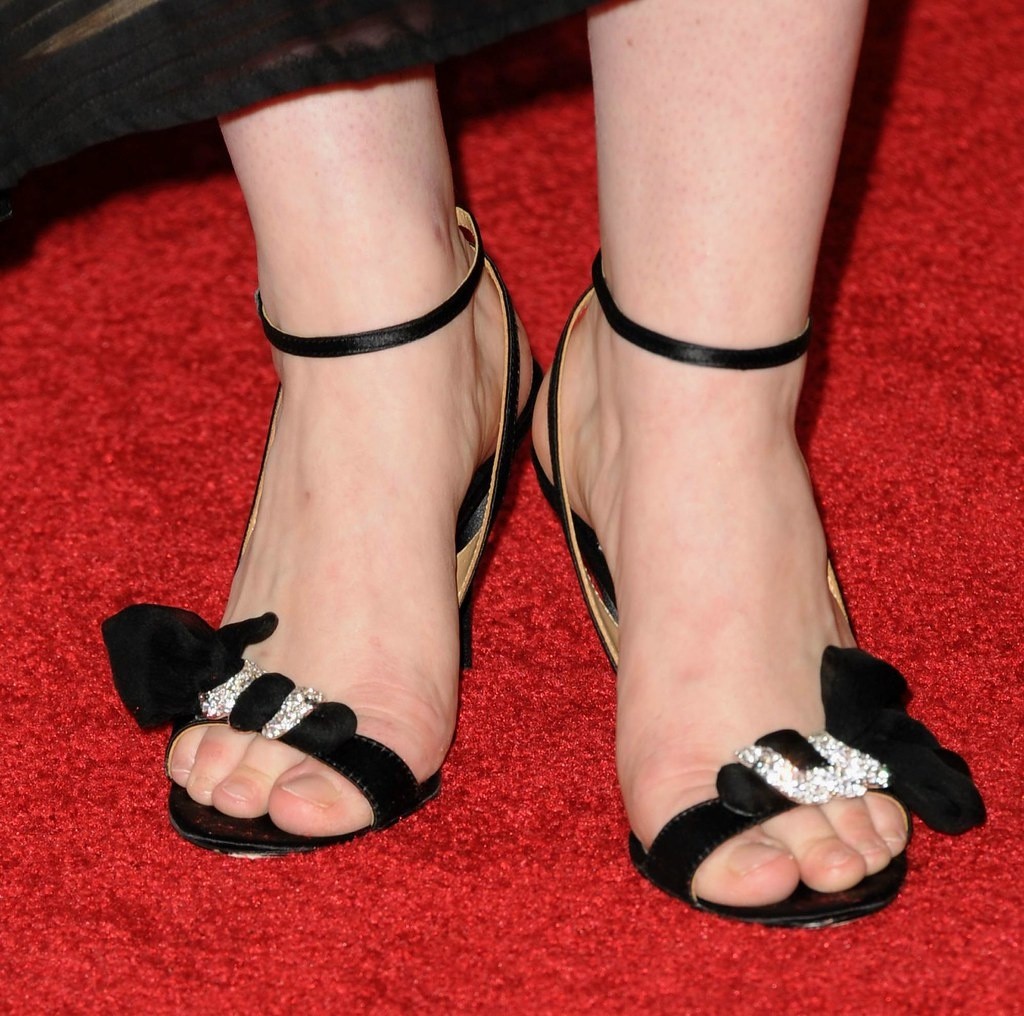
[0,0,985,927]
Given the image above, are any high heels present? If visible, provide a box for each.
[100,206,547,860]
[532,246,990,927]
